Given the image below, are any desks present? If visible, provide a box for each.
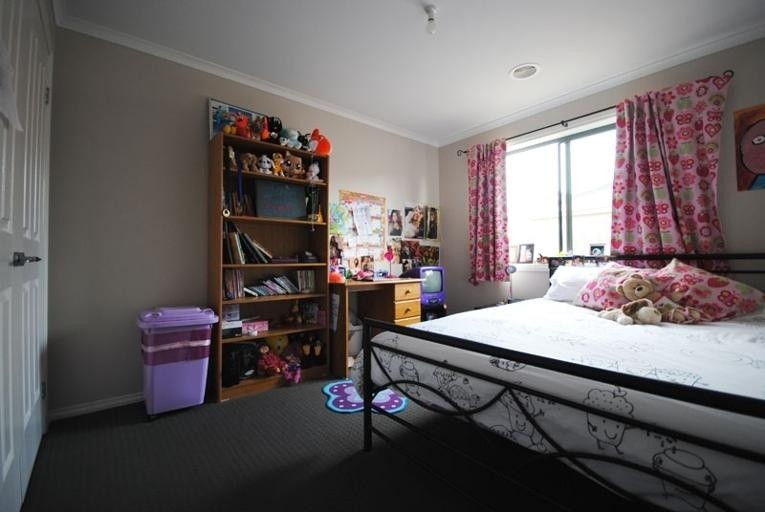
[331,277,422,377]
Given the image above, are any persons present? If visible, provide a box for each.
[390,210,402,234]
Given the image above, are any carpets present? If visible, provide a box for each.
[321,378,407,415]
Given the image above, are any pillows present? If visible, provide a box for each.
[644,257,765,322]
[544,265,604,302]
[575,262,659,311]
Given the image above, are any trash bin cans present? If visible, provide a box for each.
[347,324,365,357]
[137,305,219,419]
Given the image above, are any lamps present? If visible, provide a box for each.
[425,4,439,34]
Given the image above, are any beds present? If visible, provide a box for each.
[360,249,765,512]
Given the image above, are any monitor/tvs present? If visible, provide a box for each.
[398,266,446,306]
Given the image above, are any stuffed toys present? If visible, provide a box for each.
[225,145,322,182]
[615,274,701,324]
[598,298,662,326]
[216,109,332,155]
[256,334,325,385]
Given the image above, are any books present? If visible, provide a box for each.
[224,220,275,265]
[224,268,316,299]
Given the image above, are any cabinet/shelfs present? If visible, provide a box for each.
[207,132,329,404]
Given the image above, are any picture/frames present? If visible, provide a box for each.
[209,97,266,139]
[589,244,606,256]
[733,102,765,192]
[518,243,535,263]
